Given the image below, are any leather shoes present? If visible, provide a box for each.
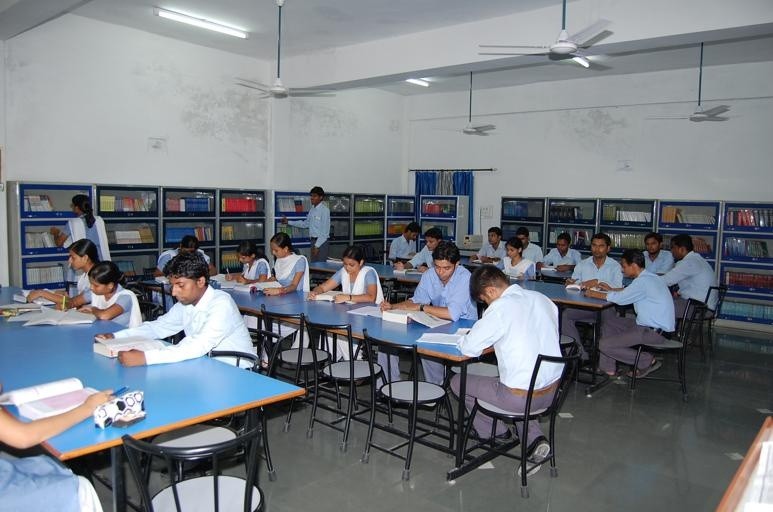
[518,435,551,478]
[496,429,512,442]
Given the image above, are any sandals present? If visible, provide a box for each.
[629,360,661,378]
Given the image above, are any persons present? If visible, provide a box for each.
[641,231,674,292]
[156,232,386,358]
[377,240,478,403]
[470,226,543,280]
[93,248,261,373]
[657,234,719,363]
[583,248,676,378]
[536,232,624,370]
[281,183,335,261]
[447,265,567,477]
[25,194,143,330]
[389,222,443,273]
[0,364,120,512]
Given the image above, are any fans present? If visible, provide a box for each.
[230,0,337,99]
[686,39,730,124]
[463,70,495,137]
[478,0,615,70]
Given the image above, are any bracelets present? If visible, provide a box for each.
[418,302,425,313]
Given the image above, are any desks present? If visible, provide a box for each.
[0,286,307,512]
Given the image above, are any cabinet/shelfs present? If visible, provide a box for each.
[499,194,772,334]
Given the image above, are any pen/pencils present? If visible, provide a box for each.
[63,296,65,310]
[226,266,229,275]
[110,386,130,396]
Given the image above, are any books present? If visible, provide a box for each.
[568,284,602,291]
[504,225,716,253]
[384,308,452,329]
[415,327,472,345]
